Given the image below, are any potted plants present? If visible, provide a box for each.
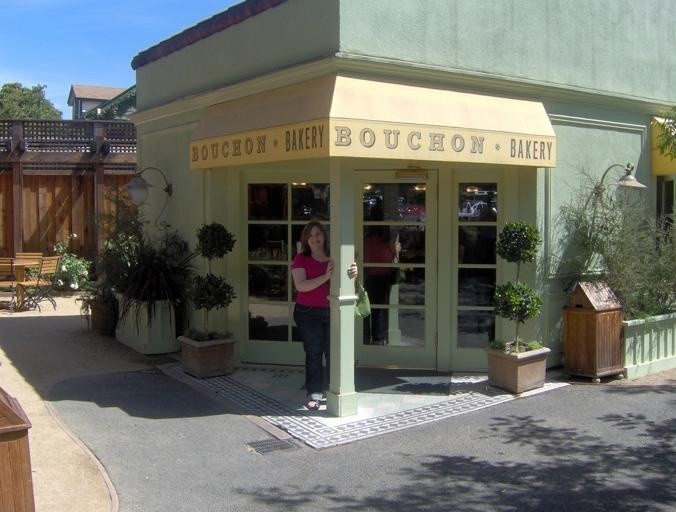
[75,183,237,379]
[485,220,551,394]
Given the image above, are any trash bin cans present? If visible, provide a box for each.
[561,280,625,383]
[0,386,37,512]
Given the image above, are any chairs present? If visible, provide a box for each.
[0,253,61,313]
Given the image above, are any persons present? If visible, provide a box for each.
[289,218,358,411]
[362,215,396,345]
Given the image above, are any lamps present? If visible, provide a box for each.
[594,162,647,195]
[125,166,172,231]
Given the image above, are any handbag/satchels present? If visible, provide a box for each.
[355,278,371,319]
[391,256,406,284]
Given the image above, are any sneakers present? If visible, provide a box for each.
[307,399,320,410]
